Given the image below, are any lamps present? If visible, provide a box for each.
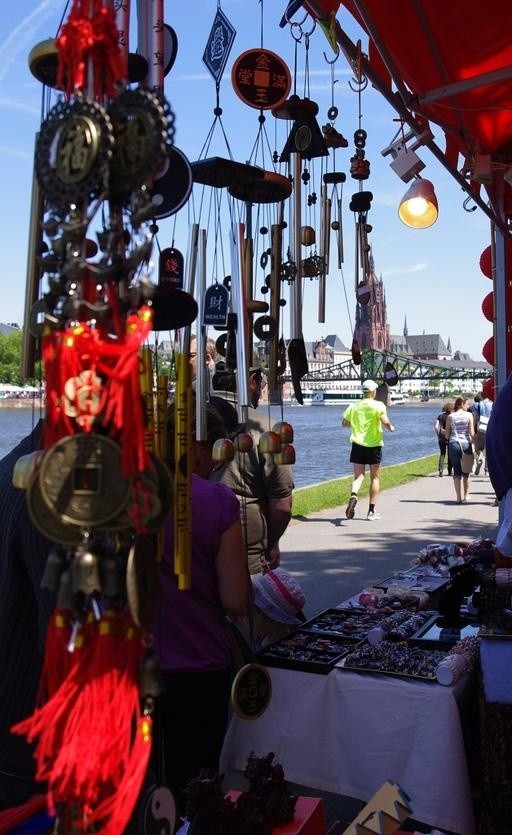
[380,113,439,230]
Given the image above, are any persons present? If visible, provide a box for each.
[434,391,495,505]
[340,378,397,520]
[485,368,512,557]
[1,332,306,835]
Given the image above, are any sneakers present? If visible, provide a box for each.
[346,495,357,518]
[367,510,380,520]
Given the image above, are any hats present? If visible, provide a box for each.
[362,380,378,392]
[250,567,305,625]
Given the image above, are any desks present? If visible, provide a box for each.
[216,545,512,834]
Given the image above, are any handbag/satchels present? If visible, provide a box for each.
[461,454,473,473]
